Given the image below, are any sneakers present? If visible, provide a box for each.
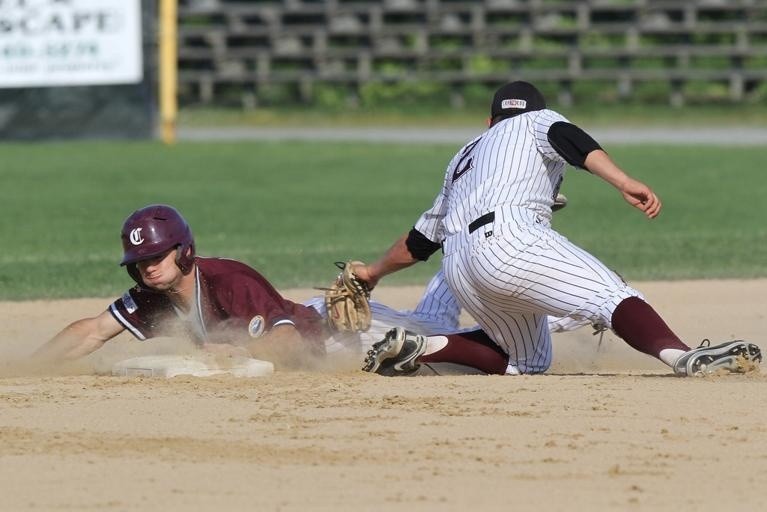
[672,335,760,379]
[361,327,425,378]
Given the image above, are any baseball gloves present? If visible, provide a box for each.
[326,261,371,332]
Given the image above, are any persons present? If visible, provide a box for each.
[328,81,763,378]
[30,191,628,378]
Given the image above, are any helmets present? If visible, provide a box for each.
[120,203,194,290]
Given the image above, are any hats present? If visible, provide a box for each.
[491,80,546,116]
